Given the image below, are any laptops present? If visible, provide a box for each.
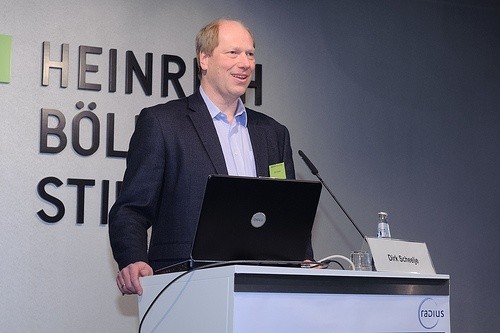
[154,174,322,275]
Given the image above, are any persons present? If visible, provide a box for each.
[108,19,321,296]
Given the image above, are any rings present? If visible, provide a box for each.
[122,284,126,288]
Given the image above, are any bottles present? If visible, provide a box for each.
[377,213,391,239]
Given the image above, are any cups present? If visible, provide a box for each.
[350,250,373,271]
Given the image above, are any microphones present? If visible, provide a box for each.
[298,150,375,271]
